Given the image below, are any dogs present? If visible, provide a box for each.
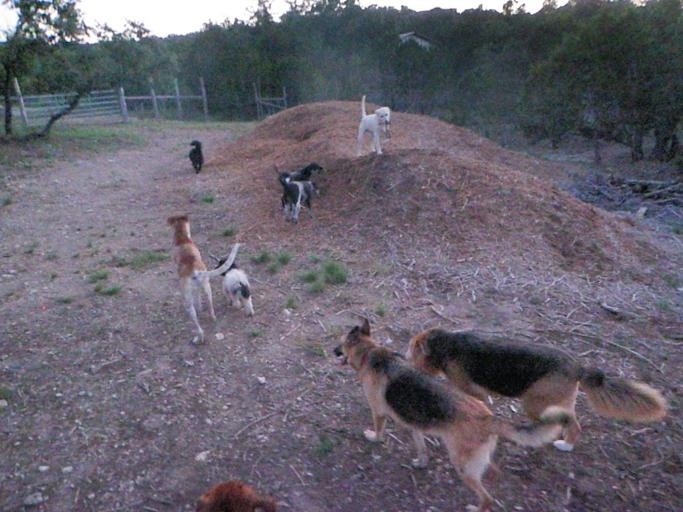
[166,213,240,348]
[277,171,320,224]
[188,140,204,173]
[275,162,323,181]
[405,324,668,453]
[196,478,275,512]
[334,316,574,512]
[214,256,254,316]
[357,94,392,160]
